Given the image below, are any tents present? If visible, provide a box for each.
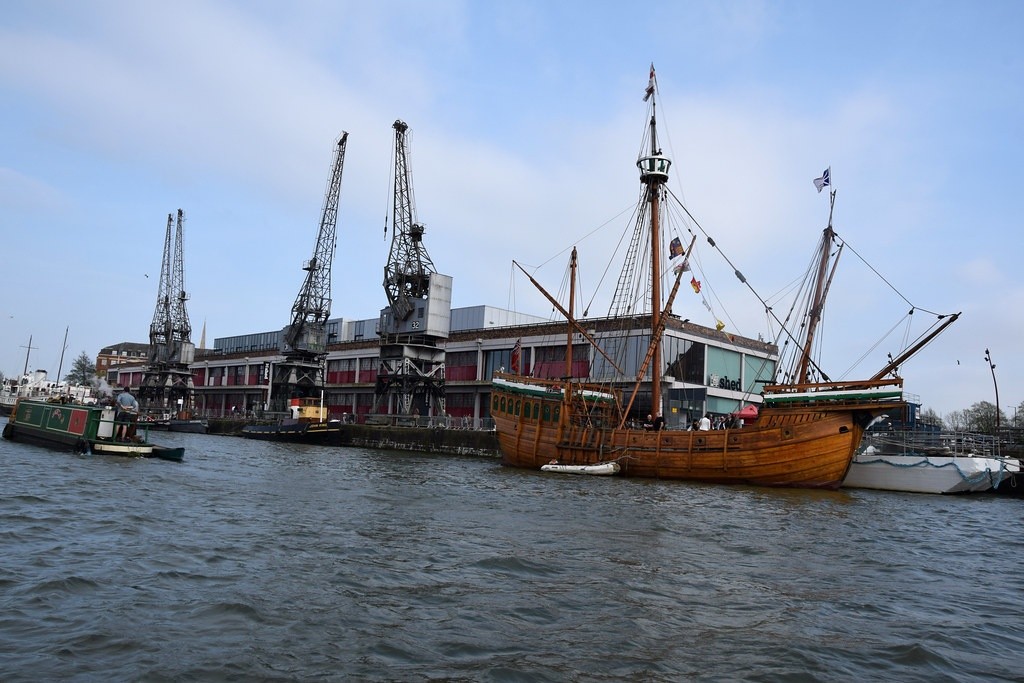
[731,405,759,419]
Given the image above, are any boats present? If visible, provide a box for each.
[844,429,1021,496]
[242,397,342,441]
[2,397,186,459]
[137,410,209,435]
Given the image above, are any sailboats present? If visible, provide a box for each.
[490,61,964,489]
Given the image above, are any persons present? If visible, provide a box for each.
[232,406,257,419]
[341,411,356,423]
[642,412,745,434]
[112,386,141,442]
[413,407,473,430]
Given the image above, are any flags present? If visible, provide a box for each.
[643,64,655,101]
[669,237,725,332]
[813,169,830,193]
[511,338,520,373]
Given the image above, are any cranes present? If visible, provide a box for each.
[370,120,453,416]
[268,131,350,415]
[136,208,195,419]
